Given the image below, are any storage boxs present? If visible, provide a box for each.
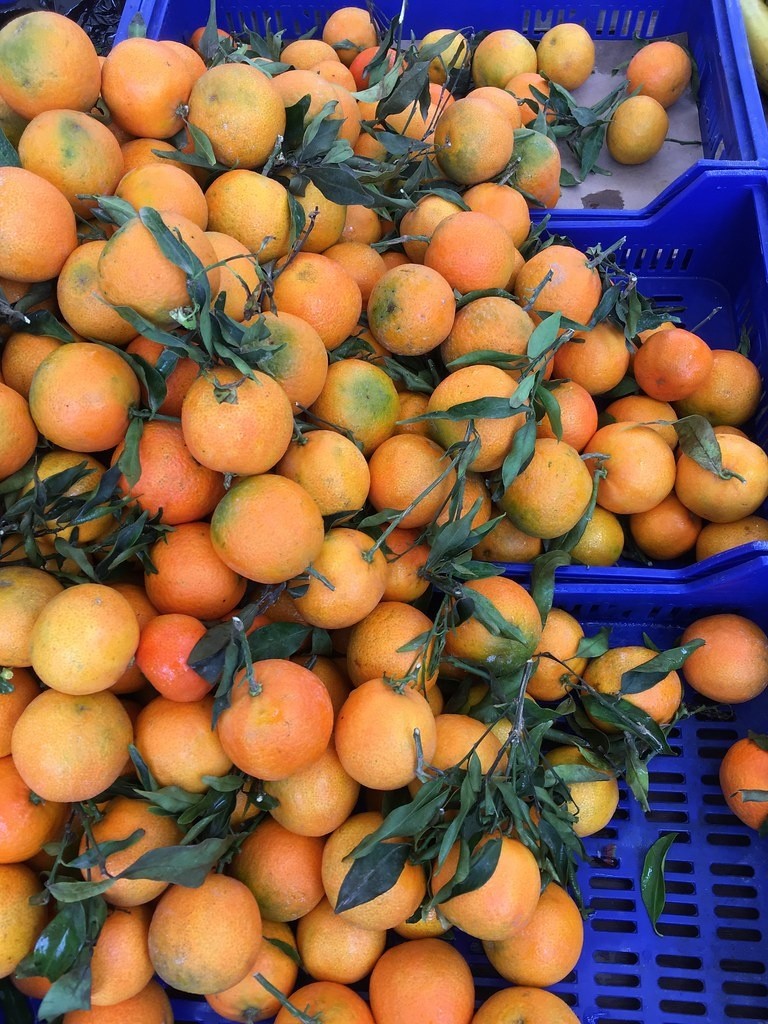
[103,0,768,1024]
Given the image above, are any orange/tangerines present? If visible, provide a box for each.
[0,8,768,1024]
